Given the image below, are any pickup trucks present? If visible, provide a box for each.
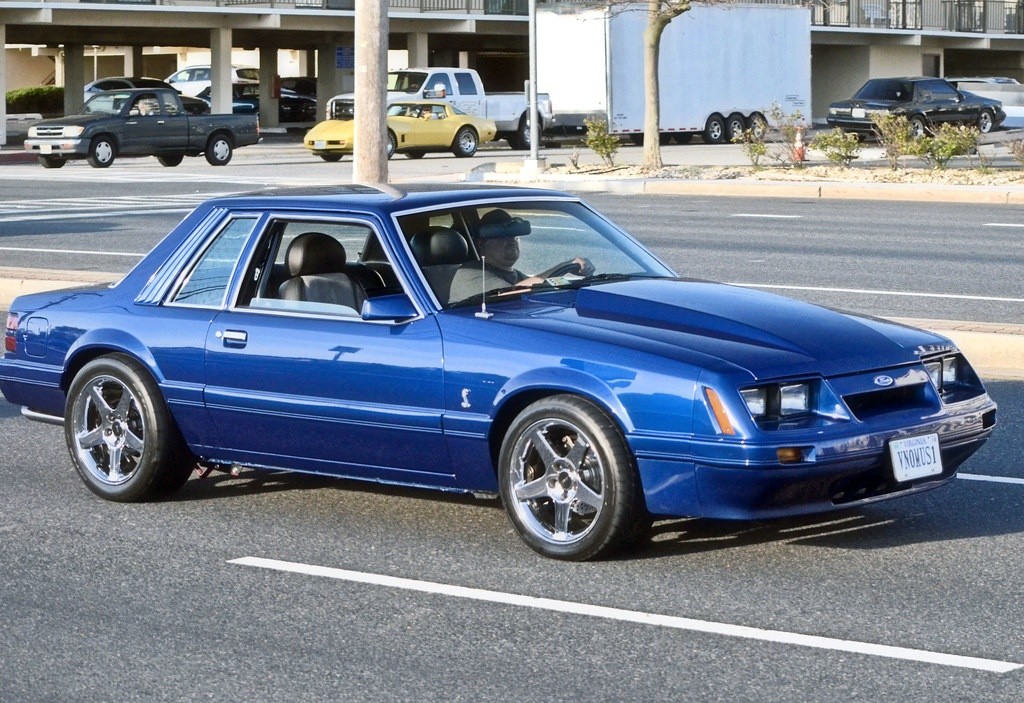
[24,87,264,169]
[326,67,555,153]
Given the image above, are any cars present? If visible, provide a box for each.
[948,77,1021,91]
[84,77,210,118]
[280,77,318,98]
[198,85,315,121]
[826,76,1007,145]
[0,180,999,565]
[302,102,498,161]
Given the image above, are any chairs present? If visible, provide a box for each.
[407,226,469,309]
[277,232,369,314]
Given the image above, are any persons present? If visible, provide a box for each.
[446,208,596,305]
[137,98,154,115]
[419,106,438,119]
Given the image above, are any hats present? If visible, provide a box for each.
[480,209,531,238]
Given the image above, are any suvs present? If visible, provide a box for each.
[162,65,261,99]
